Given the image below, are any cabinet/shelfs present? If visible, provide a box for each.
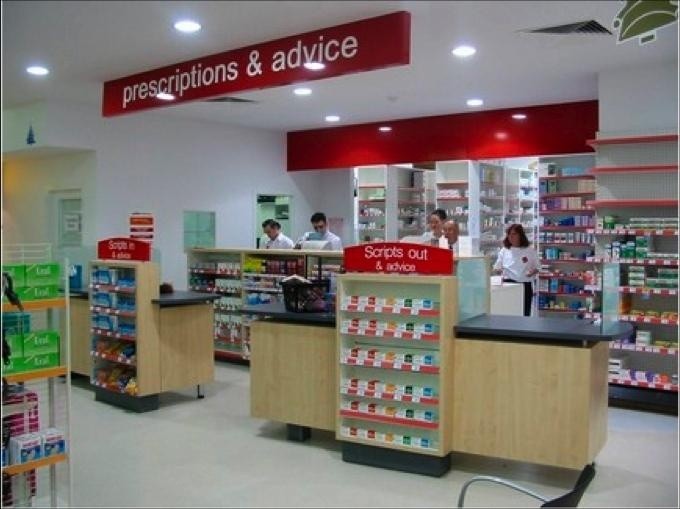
[586,130,679,418]
[86,256,161,397]
[354,158,536,279]
[332,270,456,457]
[184,244,344,367]
[532,150,600,320]
[1,238,78,508]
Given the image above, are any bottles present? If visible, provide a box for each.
[190,277,241,293]
[214,319,241,346]
[219,303,242,311]
[115,277,136,338]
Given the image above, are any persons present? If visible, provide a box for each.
[420,210,447,245]
[438,219,460,253]
[259,219,293,249]
[492,223,541,316]
[295,212,343,252]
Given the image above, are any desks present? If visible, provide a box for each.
[233,299,636,473]
[57,274,223,403]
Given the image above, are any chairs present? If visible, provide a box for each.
[454,456,601,509]
